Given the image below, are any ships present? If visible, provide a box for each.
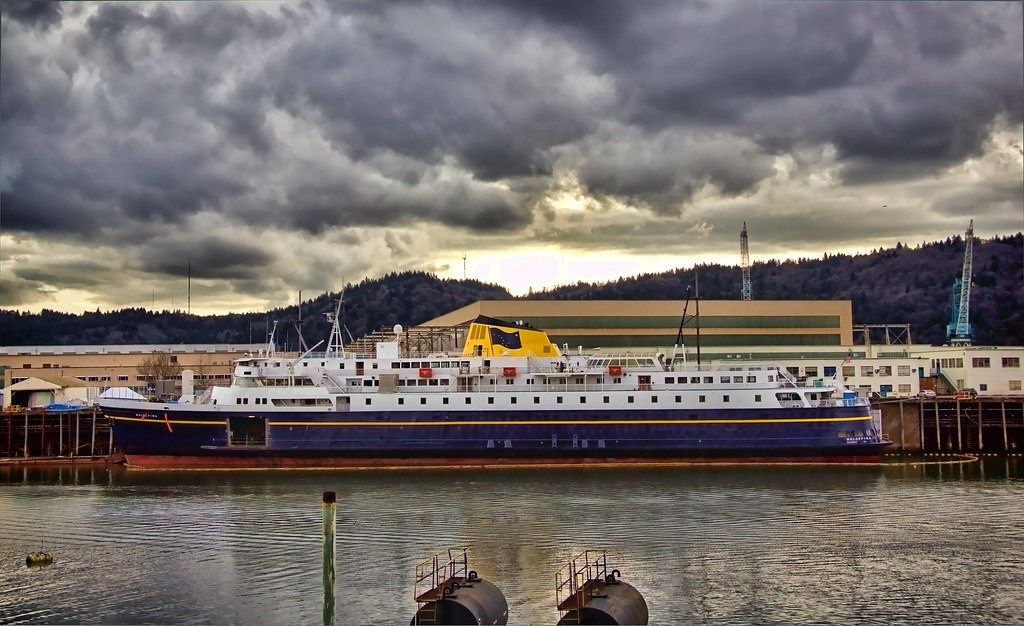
[0,282,894,485]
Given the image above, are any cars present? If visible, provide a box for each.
[917,390,936,399]
[954,388,978,398]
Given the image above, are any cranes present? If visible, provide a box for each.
[739,221,753,301]
[941,217,975,348]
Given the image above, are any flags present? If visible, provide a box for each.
[845,351,853,363]
